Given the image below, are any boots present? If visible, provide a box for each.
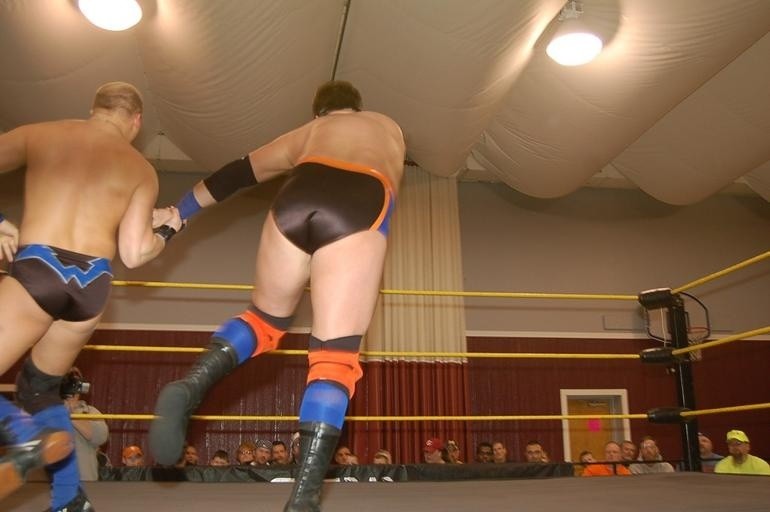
[281,420,344,512]
[146,339,238,466]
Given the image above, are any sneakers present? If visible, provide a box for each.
[0,428,94,511]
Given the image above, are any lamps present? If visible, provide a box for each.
[543,0,602,67]
[76,0,146,33]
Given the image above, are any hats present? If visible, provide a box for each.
[122,446,144,459]
[256,438,273,450]
[374,449,391,459]
[726,429,750,443]
[290,432,300,447]
[422,438,445,453]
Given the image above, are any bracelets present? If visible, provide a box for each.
[176,189,205,223]
[153,223,180,250]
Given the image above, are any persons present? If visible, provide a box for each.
[119,428,770,475]
[58,366,110,482]
[1,79,189,511]
[144,79,410,512]
